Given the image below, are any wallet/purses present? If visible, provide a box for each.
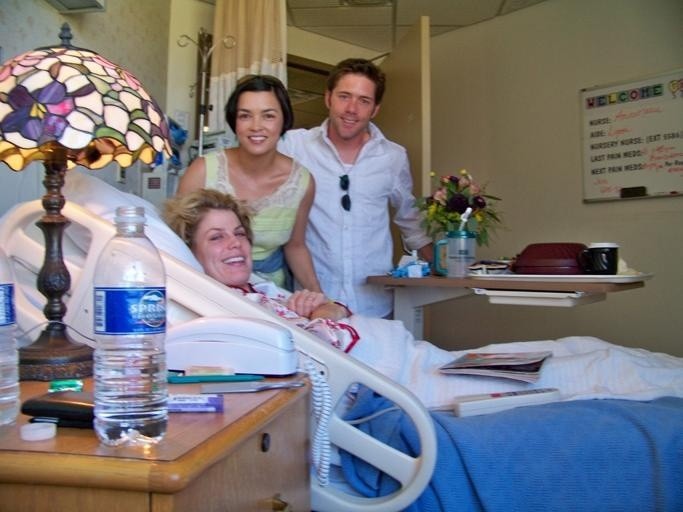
[19,389,97,427]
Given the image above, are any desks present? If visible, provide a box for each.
[366,271,656,341]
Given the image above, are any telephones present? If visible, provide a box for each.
[163,314,298,375]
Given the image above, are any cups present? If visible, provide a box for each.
[577,243,619,275]
[433,231,478,279]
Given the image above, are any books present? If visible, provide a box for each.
[438,352,551,383]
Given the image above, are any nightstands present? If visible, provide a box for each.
[0,369,315,512]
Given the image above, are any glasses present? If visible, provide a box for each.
[339,174,351,214]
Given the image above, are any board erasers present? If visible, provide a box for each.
[621,187,646,198]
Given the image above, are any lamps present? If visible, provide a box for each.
[1,21,182,381]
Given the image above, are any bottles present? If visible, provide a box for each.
[92,206,169,449]
[0,248,21,430]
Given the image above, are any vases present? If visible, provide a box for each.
[447,221,467,231]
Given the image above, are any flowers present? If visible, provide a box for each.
[411,169,502,247]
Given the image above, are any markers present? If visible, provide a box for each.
[164,393,227,415]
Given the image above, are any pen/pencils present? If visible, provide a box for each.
[166,373,266,384]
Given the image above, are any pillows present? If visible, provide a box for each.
[61,169,205,276]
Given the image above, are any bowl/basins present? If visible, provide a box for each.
[512,242,587,274]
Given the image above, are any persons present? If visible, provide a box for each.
[165,73,320,295]
[160,187,414,405]
[275,56,432,323]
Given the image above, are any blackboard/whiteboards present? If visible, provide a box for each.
[579,68,683,203]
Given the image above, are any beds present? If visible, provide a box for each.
[0,170,682,512]
